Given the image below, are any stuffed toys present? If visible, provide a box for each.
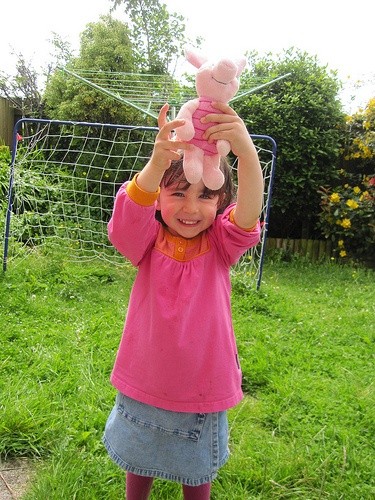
[173,42,247,191]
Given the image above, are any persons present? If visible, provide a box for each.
[96,103,270,500]
